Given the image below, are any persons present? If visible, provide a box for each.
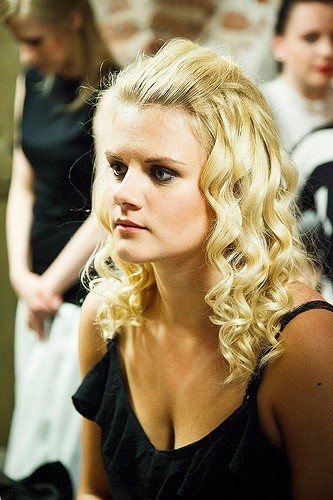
[71,38,333,500]
[0,0,333,500]
